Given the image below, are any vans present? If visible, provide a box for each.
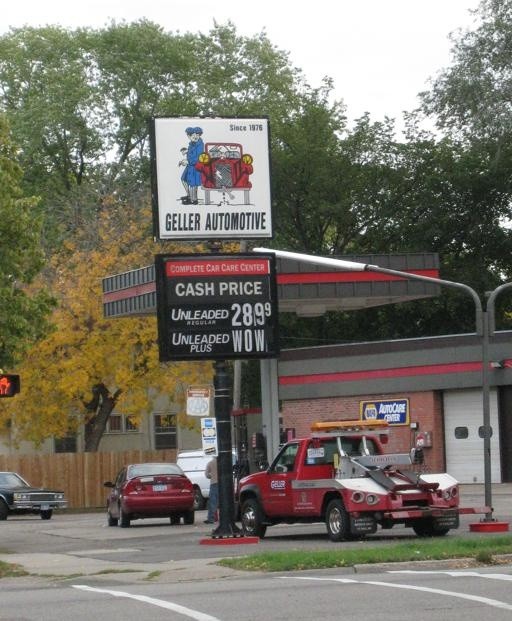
[179,449,241,509]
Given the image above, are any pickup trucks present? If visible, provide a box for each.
[233,436,461,539]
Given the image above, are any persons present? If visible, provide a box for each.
[203,456,219,524]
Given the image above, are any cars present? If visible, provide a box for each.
[0,471,65,520]
[104,463,196,528]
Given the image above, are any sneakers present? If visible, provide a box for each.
[204,520,214,524]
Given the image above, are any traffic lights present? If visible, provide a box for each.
[0,374,19,398]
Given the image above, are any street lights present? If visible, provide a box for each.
[253,246,512,532]
